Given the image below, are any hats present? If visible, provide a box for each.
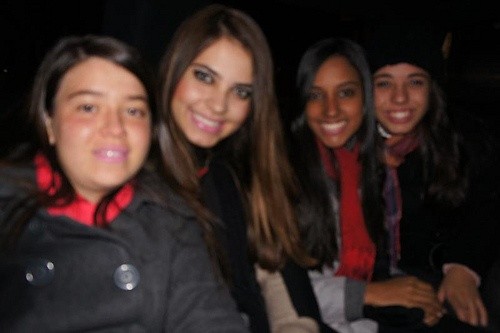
[371,37,439,76]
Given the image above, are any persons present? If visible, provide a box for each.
[365,34,500,333]
[0,32,257,333]
[141,4,339,333]
[283,37,492,333]
